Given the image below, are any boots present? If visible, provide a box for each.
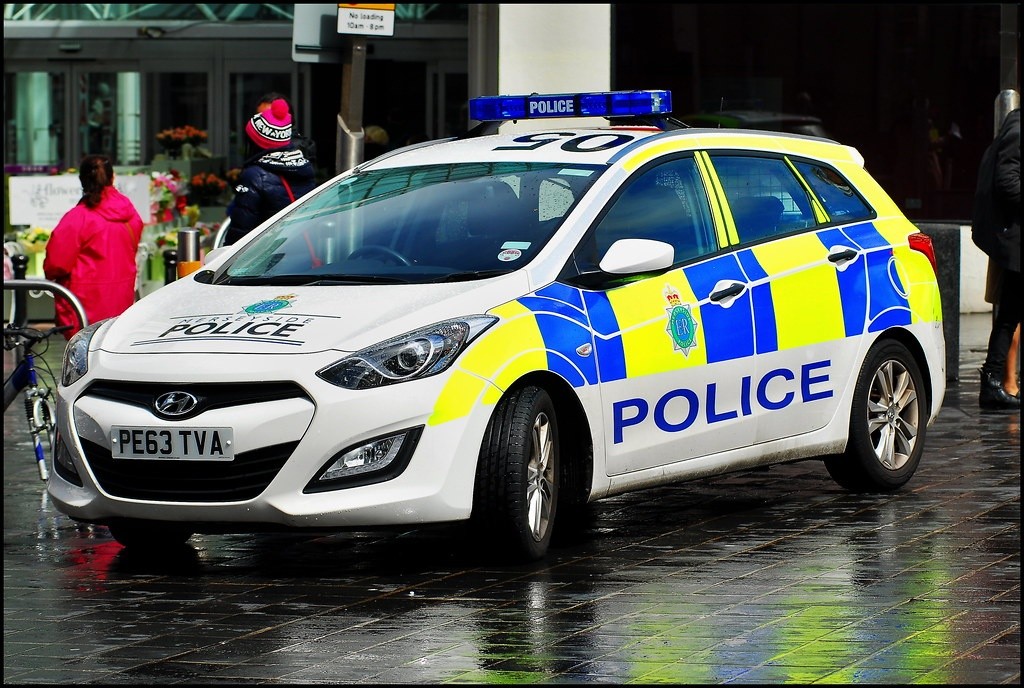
[977,368,1020,410]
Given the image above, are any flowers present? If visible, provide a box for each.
[144,128,229,250]
[17,227,52,253]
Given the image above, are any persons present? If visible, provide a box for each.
[973,107,1021,410]
[41,149,146,342]
[223,91,327,276]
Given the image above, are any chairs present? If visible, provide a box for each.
[638,186,689,250]
[436,182,526,265]
[737,195,783,244]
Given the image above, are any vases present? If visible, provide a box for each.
[26,253,45,275]
[149,252,166,281]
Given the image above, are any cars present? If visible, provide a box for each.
[50,90,949,560]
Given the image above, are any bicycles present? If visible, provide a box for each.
[3,325,74,481]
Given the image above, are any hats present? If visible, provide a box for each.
[245,98,293,151]
